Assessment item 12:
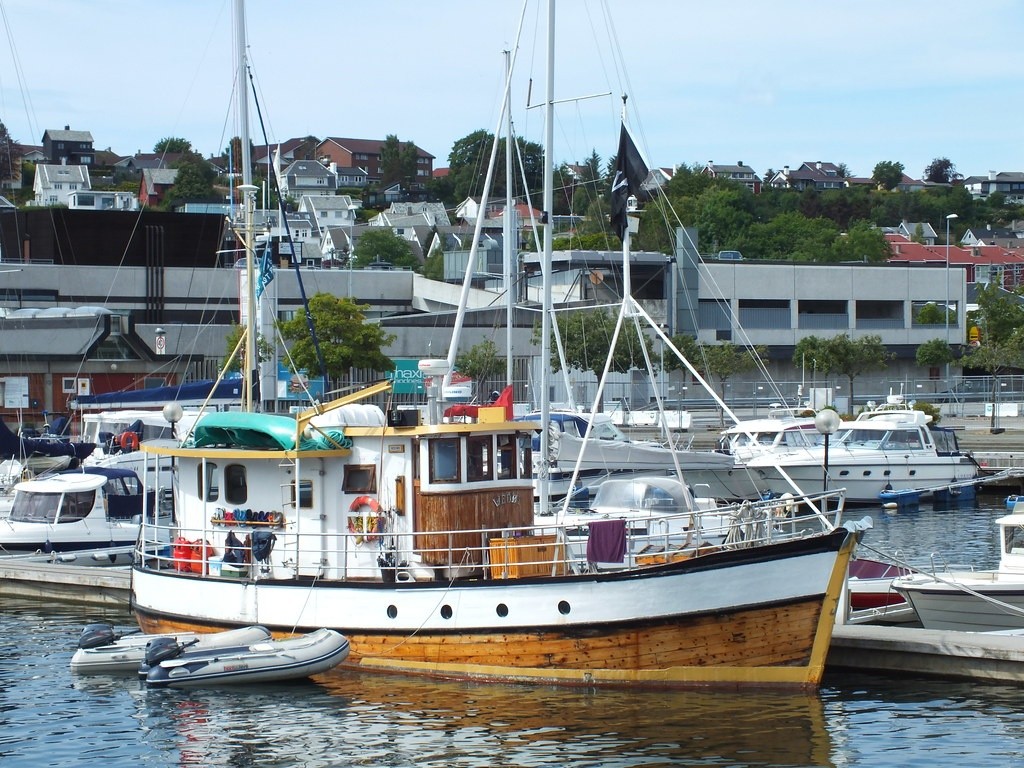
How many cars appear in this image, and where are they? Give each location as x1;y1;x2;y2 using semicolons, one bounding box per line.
715;250;747;261
934;379;995;403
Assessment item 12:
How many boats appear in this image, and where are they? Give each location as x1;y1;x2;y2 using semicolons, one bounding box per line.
0;0;1024;697
69;618;273;677
136;625;351;690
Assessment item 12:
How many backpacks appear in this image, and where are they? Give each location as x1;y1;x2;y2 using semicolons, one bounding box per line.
224;531;245;568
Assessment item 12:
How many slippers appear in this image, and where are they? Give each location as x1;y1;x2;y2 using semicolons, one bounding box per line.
214;507;283;530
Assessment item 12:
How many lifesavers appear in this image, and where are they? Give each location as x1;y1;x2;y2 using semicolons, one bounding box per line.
348;496;383;542
120;432;138;448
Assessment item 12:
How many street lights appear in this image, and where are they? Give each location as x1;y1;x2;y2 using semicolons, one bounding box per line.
946;213;958;418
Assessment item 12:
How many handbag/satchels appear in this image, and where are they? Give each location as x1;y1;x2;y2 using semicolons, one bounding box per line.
252;531;277;561
